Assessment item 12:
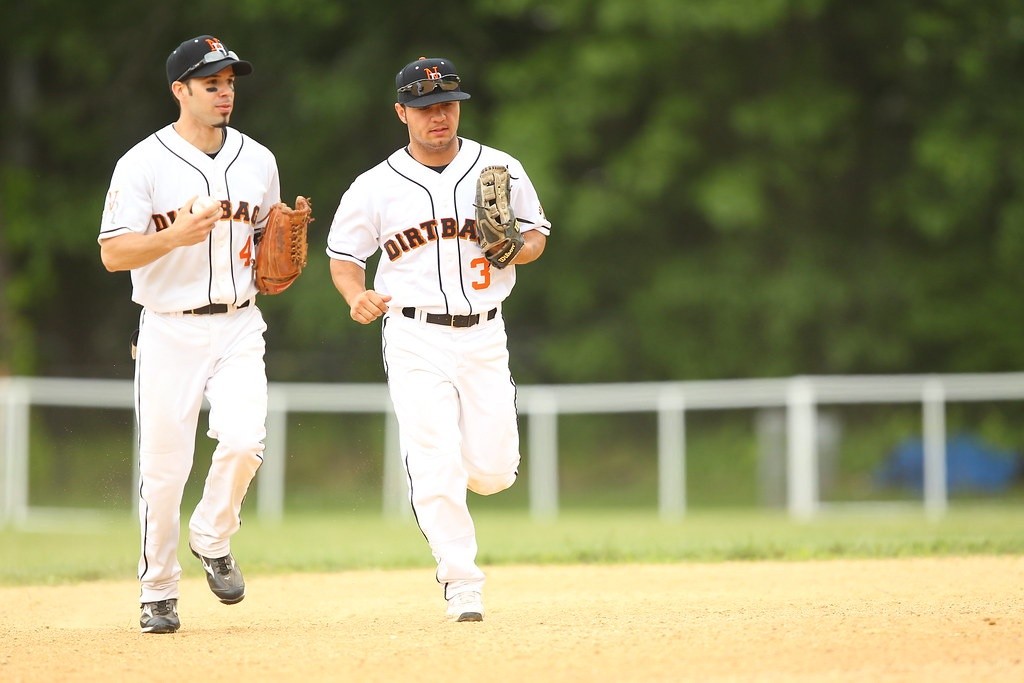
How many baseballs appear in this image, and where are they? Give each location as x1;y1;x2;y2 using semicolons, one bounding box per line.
191;195;221;220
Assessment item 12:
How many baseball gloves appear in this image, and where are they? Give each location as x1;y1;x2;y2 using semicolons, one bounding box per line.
473;164;525;271
255;195;314;296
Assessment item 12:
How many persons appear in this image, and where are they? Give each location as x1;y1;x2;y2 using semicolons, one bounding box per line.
325;56;552;631
95;36;315;635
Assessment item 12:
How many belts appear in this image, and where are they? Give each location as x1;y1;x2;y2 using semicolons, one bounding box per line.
401;306;498;328
182;298;251;315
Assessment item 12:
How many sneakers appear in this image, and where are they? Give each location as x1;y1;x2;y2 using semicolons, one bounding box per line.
138;591;181;635
189;538;248;606
443;578;489;624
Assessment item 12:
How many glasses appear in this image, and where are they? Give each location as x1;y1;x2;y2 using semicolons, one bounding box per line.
397;74;461;96
176;50;241;82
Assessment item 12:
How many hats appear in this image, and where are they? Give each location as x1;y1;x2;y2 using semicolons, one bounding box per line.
164;34;253;84
396;57;472;106
